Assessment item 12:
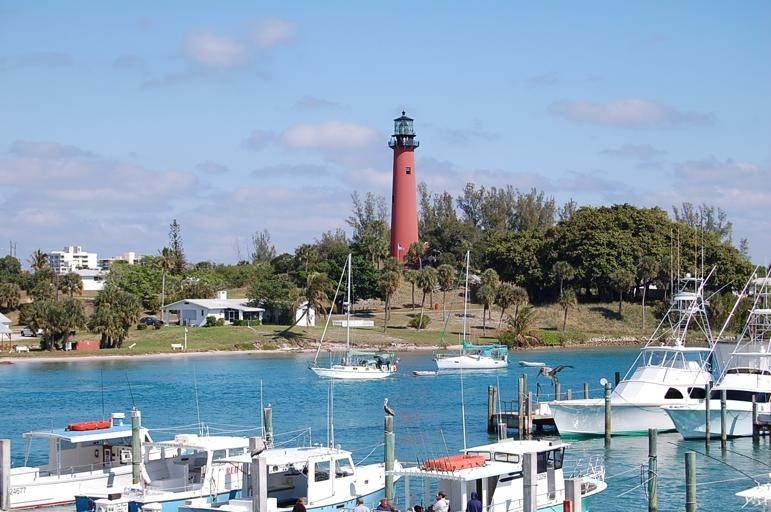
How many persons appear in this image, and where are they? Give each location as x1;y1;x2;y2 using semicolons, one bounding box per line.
466;491;482;511
292;498;306;512
376;358;383;369
413;491;450;511
354;497;371;512
377;498;393;512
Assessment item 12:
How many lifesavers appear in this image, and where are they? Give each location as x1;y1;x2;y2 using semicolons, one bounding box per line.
67;421;109;431
426;455;485;471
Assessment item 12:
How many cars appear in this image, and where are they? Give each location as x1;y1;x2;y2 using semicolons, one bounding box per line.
21;325;45;337
140;317;164;326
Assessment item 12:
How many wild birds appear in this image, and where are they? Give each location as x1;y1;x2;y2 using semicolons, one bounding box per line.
535;362;575;388
382;396;394;417
